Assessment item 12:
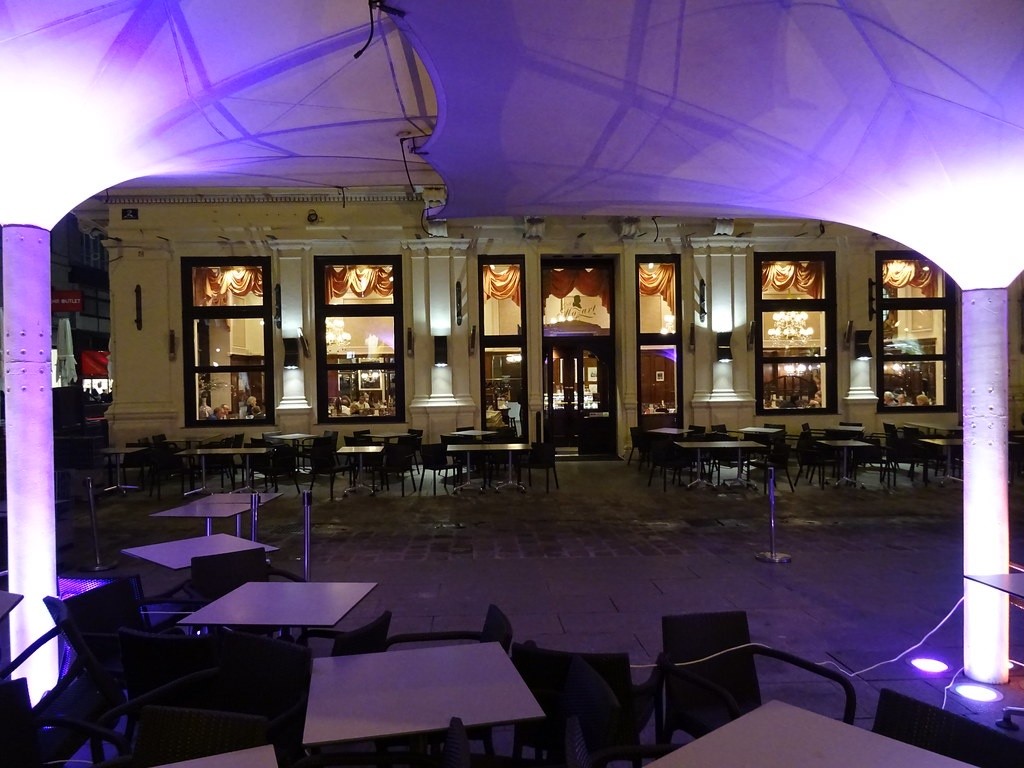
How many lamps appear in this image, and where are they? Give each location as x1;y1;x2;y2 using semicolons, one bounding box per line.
717;332;734;363
282;339;300;369
325;319;352;353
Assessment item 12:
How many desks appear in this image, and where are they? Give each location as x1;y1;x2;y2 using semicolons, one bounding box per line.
268;434;318;474
817;440;874;489
156;744;277;768
825;426;865;434
100;447;148;495
148;503;264;536
918;439;963;488
647;427;693;435
362;433;411;443
674;441;767;491
447;443;532;494
175;582;379;647
301;641;547;768
641;700;980;767
905;423;963;434
738;427;783;433
450;430;499;439
336;446;384;497
642;409;677;428
120;533;280;570
175;447;267;496
189;493;284;537
163;433;223;479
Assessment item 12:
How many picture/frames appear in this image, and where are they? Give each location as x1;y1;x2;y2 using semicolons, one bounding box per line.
358;358;383;391
587;367;597;382
337;359;357;392
559;359;577;384
656;371;664;382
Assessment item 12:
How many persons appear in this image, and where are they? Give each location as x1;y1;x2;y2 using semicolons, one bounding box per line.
206;396;266;419
83;388;113;403
327;395;370;417
763;390;822;408
884;391;929;406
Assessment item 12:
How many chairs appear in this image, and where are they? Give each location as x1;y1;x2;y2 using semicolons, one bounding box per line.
627;422;963;493
105;426;560;502
0;548;1024;767
